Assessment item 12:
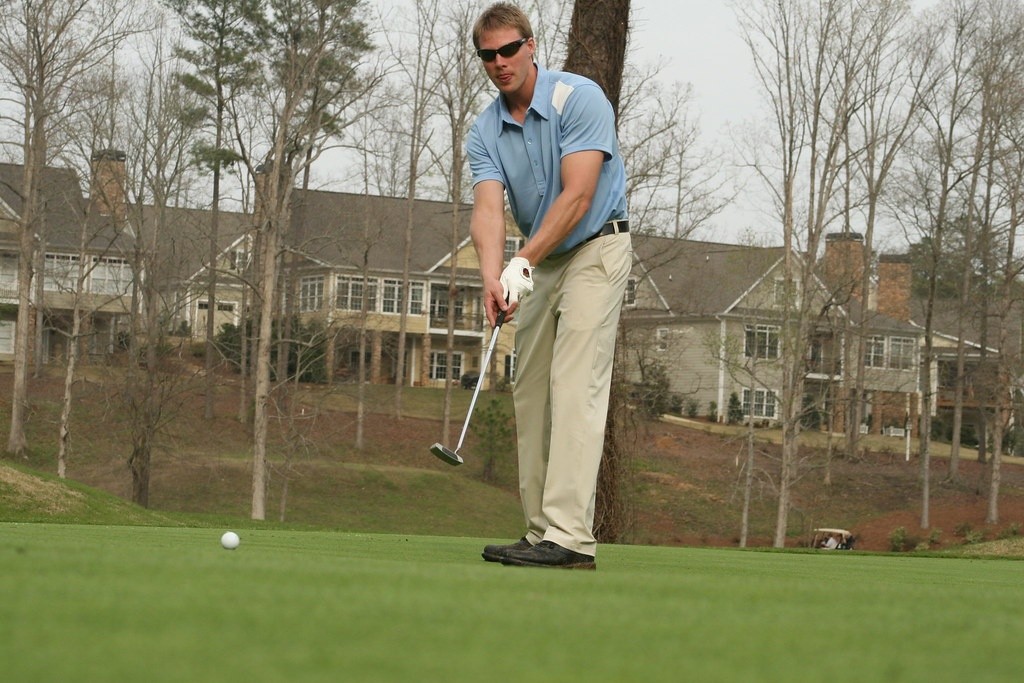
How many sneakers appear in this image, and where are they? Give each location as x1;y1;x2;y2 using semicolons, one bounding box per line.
481;536;533;562
499;540;596;570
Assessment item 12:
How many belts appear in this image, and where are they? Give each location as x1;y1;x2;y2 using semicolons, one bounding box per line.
586;220;629;242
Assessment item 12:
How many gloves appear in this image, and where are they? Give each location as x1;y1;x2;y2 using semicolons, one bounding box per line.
499;257;535;307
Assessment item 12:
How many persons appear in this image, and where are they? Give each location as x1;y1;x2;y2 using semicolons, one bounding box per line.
821;533;837;550
465;1;634;570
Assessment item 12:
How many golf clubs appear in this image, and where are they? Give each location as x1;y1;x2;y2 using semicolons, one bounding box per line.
430;293;511;465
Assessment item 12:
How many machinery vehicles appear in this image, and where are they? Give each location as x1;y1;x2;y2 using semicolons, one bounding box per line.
812;528;853;550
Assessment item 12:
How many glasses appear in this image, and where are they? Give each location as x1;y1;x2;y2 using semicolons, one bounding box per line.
477;37;527;62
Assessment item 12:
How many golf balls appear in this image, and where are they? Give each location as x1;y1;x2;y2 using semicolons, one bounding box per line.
221;531;240;550
